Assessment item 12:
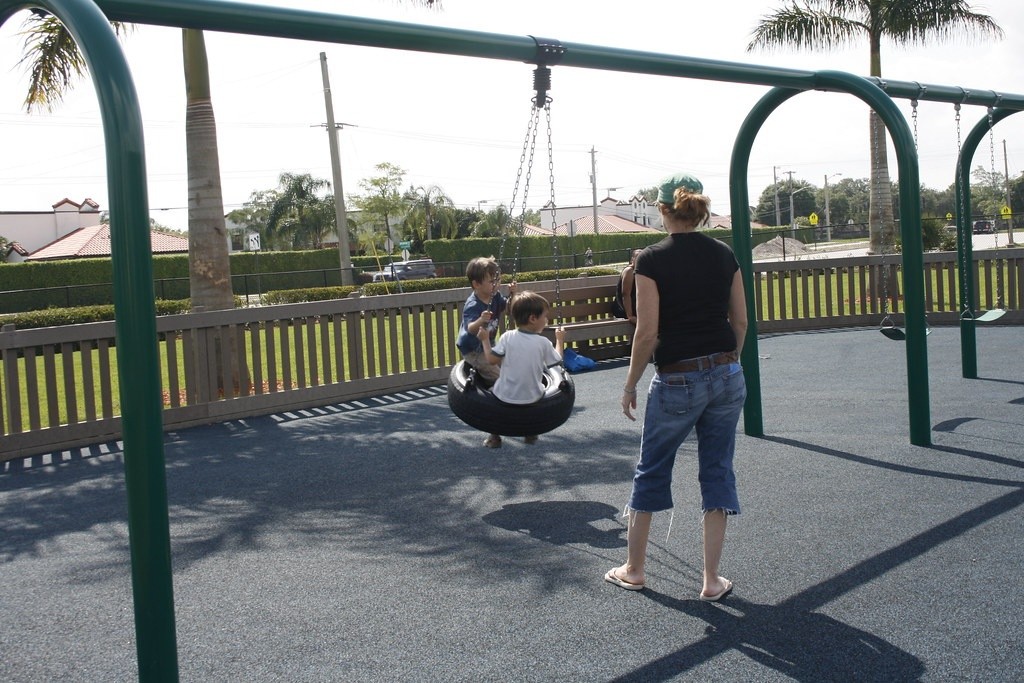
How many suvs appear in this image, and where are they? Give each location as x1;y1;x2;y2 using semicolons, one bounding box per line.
972;221;994;235
373;259;437;282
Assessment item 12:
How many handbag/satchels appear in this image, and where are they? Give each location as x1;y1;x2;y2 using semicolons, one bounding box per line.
562;348;596;372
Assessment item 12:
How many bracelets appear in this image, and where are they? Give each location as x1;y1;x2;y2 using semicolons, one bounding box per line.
621;382;638;393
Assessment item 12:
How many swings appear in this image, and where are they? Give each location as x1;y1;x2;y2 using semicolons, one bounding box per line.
446;63;576;438
868;97;933;342
954;102;1009;323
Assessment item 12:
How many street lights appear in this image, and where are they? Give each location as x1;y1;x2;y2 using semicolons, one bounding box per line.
823;172;842;242
478;200;487;212
788;185;809;239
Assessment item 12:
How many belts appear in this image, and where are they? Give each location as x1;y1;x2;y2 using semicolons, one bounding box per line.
659;350;738;372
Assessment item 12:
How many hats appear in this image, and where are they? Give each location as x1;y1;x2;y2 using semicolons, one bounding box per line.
657;172;703;204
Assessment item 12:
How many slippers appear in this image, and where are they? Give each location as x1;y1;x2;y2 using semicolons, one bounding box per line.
700;577;732;601
605;568;643;589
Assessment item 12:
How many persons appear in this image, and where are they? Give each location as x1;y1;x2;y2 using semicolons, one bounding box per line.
604;171;747;600
609;248;642;326
454;254;518;383
584;246;593;265
474;289;566;448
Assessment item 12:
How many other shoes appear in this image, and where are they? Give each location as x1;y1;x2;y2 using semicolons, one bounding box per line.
523;435;538;445
483;435;502;448
